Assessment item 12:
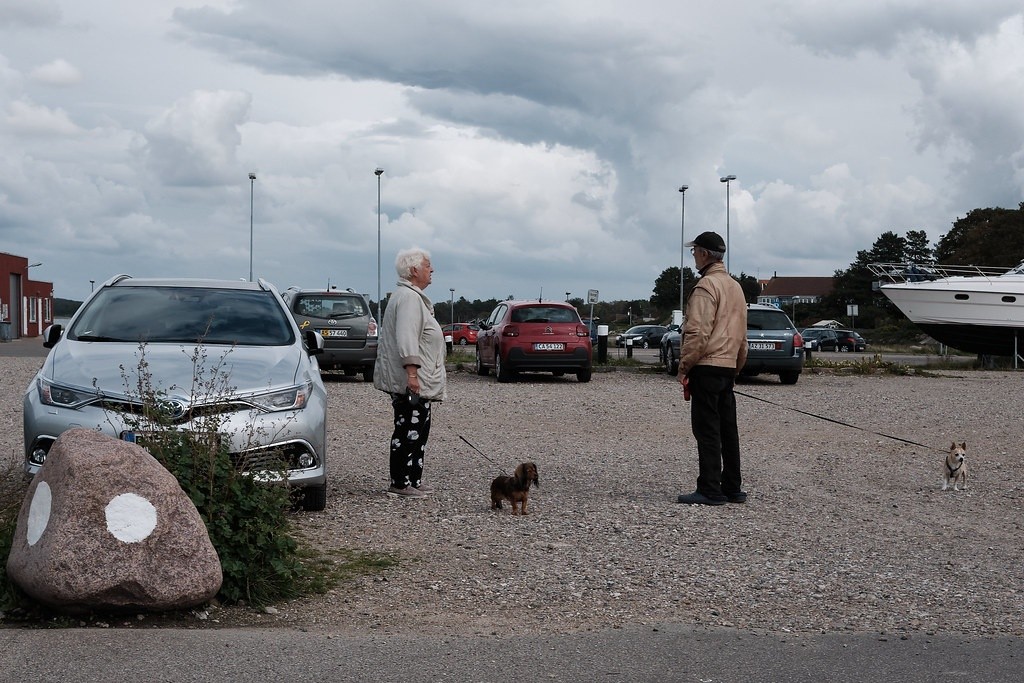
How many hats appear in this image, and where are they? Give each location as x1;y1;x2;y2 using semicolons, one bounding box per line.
684;232;726;253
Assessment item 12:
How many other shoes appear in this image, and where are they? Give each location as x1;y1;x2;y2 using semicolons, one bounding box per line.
417;484;434;491
724;494;746;502
678;492;725;505
388;484;424;496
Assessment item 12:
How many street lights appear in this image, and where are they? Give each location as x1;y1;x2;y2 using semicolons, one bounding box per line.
88;280;95;295
375;166;386;336
720;175;737;276
248;172;256;282
678;184;688;310
20;262;42;337
449;287;456;354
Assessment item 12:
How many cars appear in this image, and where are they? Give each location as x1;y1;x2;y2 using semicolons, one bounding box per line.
475;298;598;383
23;274;327;511
661;302;805;386
616;325;669;350
442;323;482;345
799;328;866;352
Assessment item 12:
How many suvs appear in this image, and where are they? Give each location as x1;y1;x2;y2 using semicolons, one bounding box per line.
277;286;378;382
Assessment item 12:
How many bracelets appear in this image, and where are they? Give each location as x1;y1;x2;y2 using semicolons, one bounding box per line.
409;375;417;378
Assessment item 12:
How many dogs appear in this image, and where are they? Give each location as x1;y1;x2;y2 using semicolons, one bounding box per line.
941;441;968;492
490;462;539;516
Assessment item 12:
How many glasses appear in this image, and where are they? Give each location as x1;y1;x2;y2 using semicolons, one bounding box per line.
690;248;700;254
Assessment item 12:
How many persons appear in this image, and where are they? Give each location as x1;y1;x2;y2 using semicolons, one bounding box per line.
373;247;446;497
675;232;748;503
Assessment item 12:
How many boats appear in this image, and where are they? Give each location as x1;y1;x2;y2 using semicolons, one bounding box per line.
867;257;1024;356
